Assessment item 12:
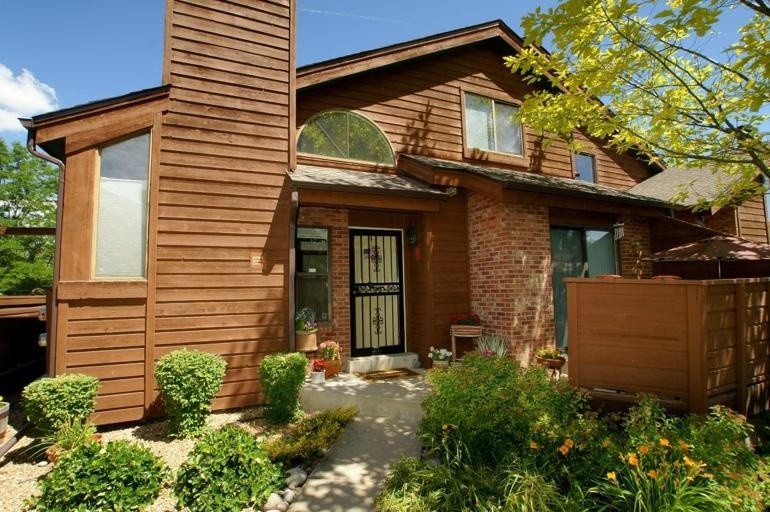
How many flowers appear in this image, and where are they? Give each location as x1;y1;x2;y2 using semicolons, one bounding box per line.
313;360;326;372
452;314;479;325
296;308;319;332
428;346;452;361
318;340;341;360
537;347;561;357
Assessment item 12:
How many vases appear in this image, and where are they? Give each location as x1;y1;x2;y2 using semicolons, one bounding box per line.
432;360;449;369
295;329;317;353
311;372;326;384
536;358;565;370
323;360;341;377
